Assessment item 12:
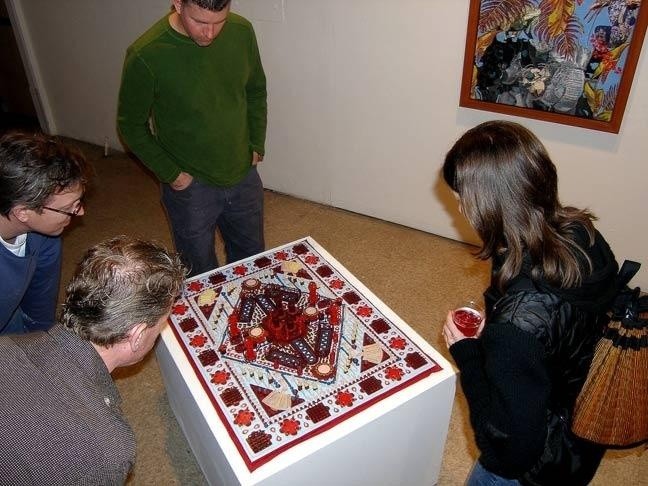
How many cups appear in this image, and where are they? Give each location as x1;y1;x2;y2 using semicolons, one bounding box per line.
452;302;484;337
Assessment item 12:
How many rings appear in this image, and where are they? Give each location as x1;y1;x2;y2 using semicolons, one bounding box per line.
440;330;446;336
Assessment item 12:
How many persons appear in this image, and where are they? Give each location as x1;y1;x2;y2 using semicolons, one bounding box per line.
0;131;95;337
1;235;191;486
440;117;647;486
113;0;270;277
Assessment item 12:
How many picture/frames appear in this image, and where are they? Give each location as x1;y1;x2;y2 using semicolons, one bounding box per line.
458;0;648;135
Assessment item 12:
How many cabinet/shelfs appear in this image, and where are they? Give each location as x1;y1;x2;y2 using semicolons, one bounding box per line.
154;236;459;486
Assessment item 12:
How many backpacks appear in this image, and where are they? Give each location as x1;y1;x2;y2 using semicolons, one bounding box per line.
531;227;648;451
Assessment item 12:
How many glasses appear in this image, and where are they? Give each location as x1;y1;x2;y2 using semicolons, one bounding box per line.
40;201;83;219
